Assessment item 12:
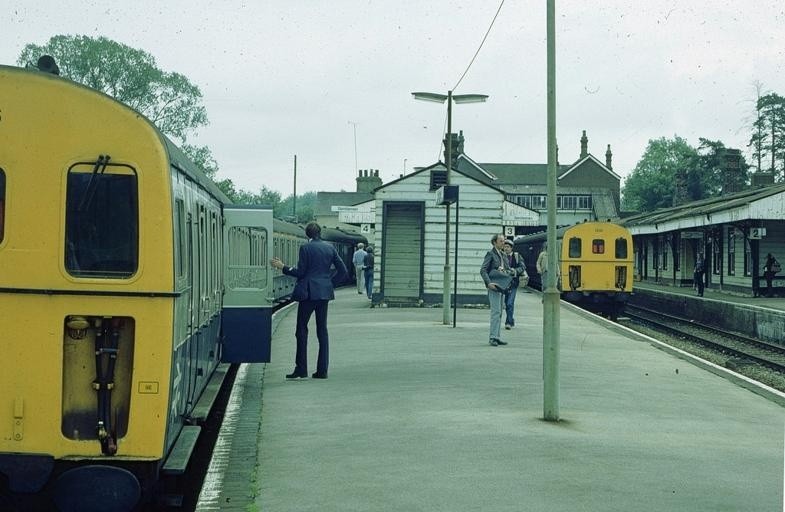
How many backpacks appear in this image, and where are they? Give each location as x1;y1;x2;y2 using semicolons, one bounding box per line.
514;252;530;289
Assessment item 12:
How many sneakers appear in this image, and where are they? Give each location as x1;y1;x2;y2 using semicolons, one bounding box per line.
505;322;514;331
489;339;508;346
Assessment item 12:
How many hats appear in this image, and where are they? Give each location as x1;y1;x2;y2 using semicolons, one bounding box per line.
505;240;514;246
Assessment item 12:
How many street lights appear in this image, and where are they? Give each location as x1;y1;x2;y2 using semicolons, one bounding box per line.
407;85;494;324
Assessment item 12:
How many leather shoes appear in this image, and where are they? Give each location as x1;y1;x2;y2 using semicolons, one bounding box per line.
285;369;308;379
312;371;330;379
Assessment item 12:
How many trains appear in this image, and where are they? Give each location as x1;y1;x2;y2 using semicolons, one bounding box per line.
1;48;379;512
512;217;637;321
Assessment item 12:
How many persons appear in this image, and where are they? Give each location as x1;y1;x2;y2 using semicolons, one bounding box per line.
266;222;347;379
535;241;559;303
351;241;368;294
762;252;780;295
501;238;528;329
479;233;508;347
694;252;708;298
364;246;375;300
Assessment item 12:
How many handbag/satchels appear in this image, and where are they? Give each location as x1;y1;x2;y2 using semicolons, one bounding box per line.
486;269;514;294
